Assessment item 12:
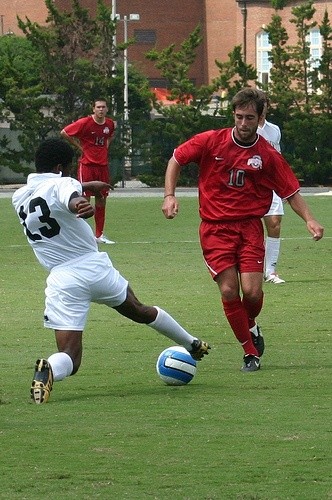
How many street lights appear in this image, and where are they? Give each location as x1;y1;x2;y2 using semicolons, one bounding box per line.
111;13;141;172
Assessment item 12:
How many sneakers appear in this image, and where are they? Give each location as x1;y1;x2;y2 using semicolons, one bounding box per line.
243;354;260;372
248;321;264;357
187;338;211;361
95;234;115;245
30;359;53;405
265;273;285;284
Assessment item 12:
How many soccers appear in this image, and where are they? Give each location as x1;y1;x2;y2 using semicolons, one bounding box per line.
156;346;196;385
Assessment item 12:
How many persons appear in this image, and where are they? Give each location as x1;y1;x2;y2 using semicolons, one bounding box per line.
60;98;116;245
12;140;211;405
162;88;325;371
256;96;285;284
